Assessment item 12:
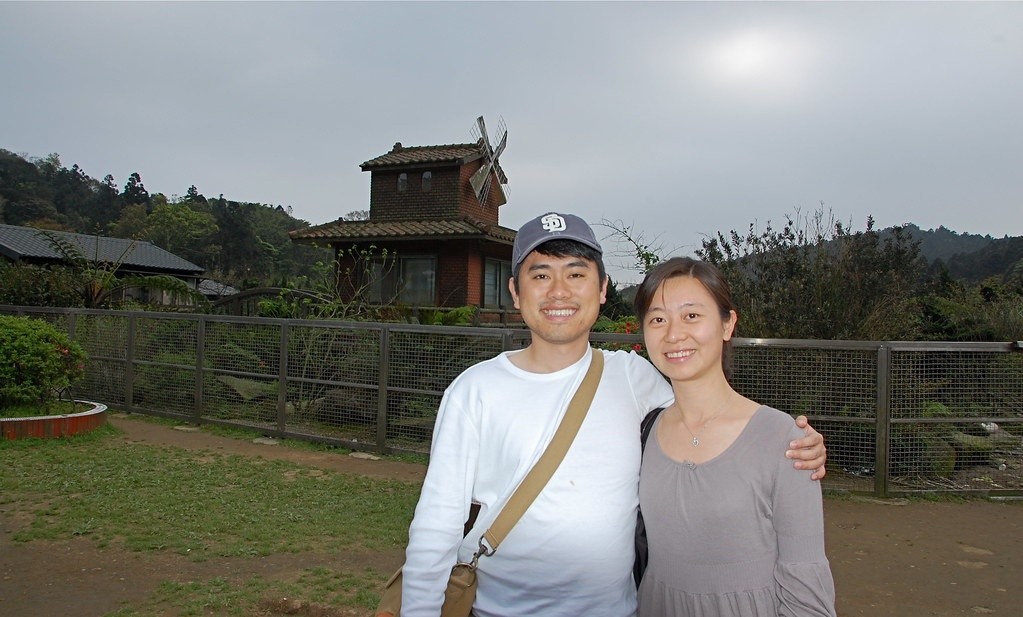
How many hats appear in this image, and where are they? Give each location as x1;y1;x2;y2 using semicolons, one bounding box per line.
512;212;604;275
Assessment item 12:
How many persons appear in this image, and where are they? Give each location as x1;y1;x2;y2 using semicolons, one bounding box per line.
397;212;825;617
637;257;837;617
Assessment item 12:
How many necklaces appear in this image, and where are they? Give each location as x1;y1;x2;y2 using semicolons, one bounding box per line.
673;405;723;447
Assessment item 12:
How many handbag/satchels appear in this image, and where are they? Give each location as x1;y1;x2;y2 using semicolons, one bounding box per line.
633;407;665;592
375;548;478;617
